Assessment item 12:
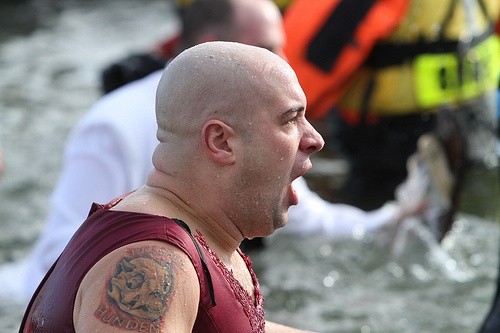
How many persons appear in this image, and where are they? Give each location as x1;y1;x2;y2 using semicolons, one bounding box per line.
149;1;500;243
30;0;289;296
19;40;325;333
